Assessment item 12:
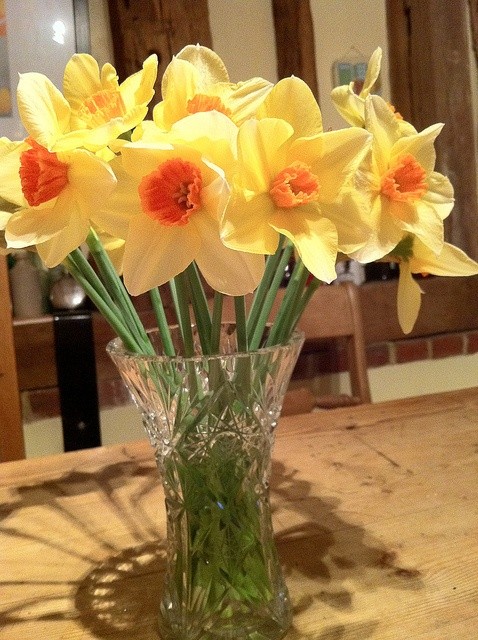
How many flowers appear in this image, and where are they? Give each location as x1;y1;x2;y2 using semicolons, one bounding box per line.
0;43;478;627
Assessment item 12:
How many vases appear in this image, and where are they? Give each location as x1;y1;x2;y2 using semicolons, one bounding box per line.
107;325;303;639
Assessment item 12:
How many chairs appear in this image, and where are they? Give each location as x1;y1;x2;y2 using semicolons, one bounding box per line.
186;278;372;418
9;310;102;452
0;249;26;463
